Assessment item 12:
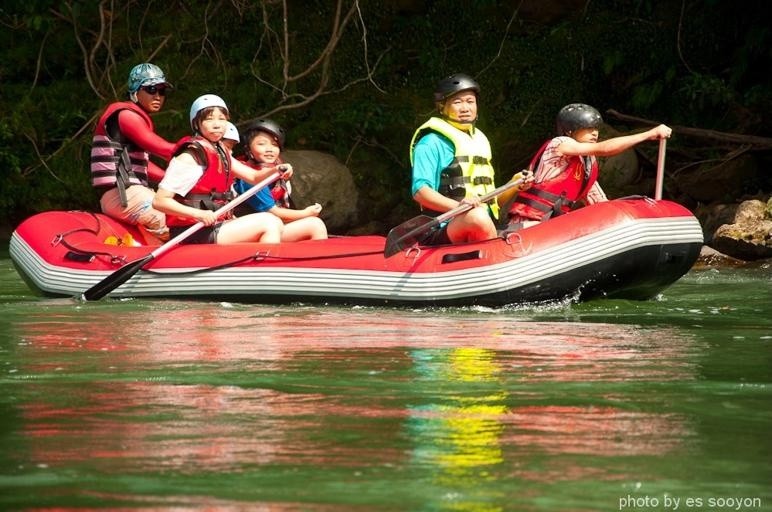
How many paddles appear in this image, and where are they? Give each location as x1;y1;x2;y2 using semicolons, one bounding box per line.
383;169;529;258
82;165;289;303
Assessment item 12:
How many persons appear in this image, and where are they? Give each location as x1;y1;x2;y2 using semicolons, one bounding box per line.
232;117;323;224
90;63;177;242
409;71;534;247
507;102;673;229
151;93;329;244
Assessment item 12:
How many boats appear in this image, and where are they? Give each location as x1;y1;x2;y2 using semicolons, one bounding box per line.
8;193;705;309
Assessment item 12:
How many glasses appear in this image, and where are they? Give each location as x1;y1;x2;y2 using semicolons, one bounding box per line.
139;86;166;96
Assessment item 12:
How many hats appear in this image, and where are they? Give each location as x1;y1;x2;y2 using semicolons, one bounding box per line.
222;120;241;143
435;74;478;99
560;104;603;132
248;118;286;147
192;95;227;129
127;63;173;92
